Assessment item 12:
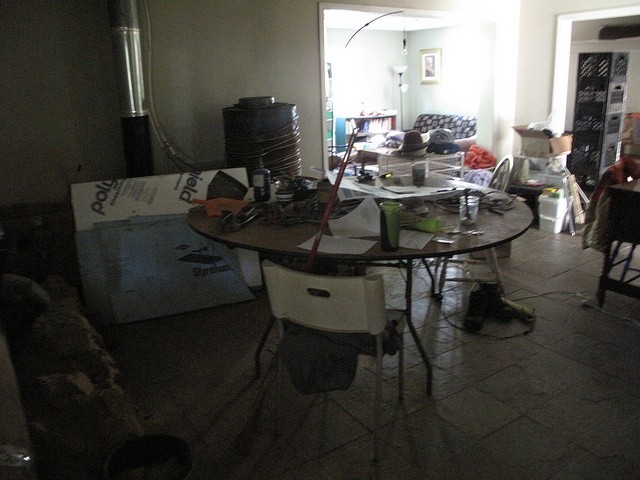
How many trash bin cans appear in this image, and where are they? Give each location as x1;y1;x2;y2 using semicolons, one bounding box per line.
102;433;192;480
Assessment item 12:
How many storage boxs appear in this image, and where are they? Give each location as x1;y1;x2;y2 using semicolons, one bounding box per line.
529;155;567;173
538;196;571;234
512;124;573;157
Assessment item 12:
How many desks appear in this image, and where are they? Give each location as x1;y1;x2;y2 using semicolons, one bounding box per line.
596;176;640;310
353;142;465;178
186;171;534;376
343;108;397;131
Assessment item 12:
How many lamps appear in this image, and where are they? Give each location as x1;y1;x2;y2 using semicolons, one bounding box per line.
393;65;409;132
401;27;407;56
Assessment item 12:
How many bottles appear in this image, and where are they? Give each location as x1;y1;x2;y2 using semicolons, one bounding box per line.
253;163;271;200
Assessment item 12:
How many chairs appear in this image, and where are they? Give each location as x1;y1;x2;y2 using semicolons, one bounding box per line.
261;260;406;463
430;155;517;296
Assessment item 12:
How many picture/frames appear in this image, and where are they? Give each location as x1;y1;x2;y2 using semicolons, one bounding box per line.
418;49;442;83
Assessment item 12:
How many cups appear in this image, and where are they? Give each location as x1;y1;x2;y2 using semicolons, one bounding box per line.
379;201;403;251
412;158;428;187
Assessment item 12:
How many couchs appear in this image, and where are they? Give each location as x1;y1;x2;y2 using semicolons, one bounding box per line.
403;114;477;153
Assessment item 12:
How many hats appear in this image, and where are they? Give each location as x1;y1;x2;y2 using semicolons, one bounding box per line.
392;132;429;153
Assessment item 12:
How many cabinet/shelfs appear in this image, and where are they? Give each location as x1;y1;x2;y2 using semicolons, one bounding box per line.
325;62;336;157
570;51;628;190
514;185;552;229
